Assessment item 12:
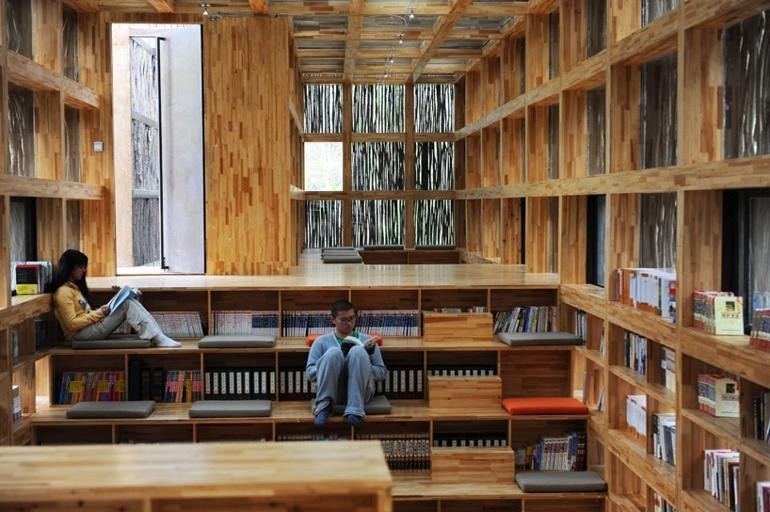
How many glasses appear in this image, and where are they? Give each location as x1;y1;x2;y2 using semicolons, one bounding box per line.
334;316;358;324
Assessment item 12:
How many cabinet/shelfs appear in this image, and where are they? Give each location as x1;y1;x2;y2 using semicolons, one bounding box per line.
128;34;170;272
453;0;770;512
0;0;116;294
303;77;456;251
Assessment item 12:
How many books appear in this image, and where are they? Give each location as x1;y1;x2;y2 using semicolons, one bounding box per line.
352;306;556;401
12;330;23;422
16;261;53;295
595;265;770;511
274;431;585;474
59;310;334;404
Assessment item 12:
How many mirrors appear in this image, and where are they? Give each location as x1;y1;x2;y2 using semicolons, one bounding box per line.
111;22;206;277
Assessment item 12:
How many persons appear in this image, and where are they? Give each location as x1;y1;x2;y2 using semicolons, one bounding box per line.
45;248;182;348
